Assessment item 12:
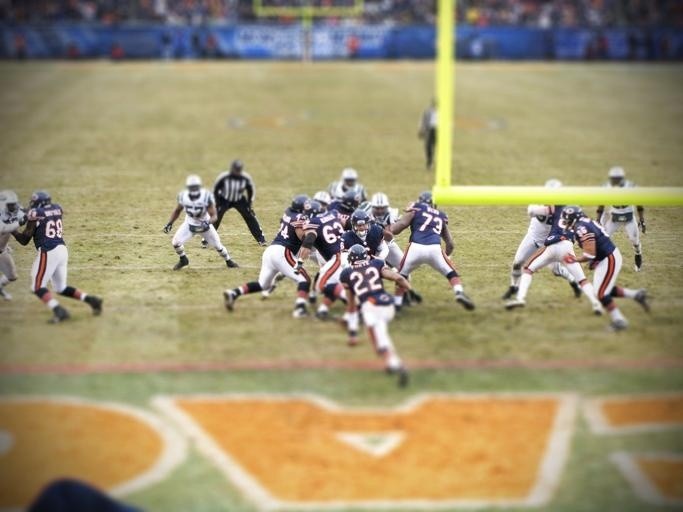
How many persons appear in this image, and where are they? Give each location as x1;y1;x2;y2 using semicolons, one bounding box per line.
0;190;43;302
501;166;657;332
10;190;104;325
415;97;439;169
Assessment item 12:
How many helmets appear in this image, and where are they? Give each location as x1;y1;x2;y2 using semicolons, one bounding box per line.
340;167;357;181
369;191;379;207
346;242;367;268
415;191;437;208
342;190;360;207
29;190;52;210
184;174;202;198
349;209;371;237
559;205;584;229
288;190;333;218
0;188;20;217
607;165;625;179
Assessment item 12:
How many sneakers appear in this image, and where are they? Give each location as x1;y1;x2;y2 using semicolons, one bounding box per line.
200;237;209;249
45;305;72;325
171;255;189;273
83;295;103;318
632;287;653;316
568;279;581;299
454;290;475;312
592;301;627;330
0;287;13;301
257;239;267;247
500;284;526;311
224;259;239;269
291;304;328;321
223;288;237;313
632;252;643;272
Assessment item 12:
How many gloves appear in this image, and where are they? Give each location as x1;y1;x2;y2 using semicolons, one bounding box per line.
162;222;173;234
247;205;256;217
199;220;210;230
563;253;575;266
637;220;648;234
294;257;304;275
407;288;421;305
